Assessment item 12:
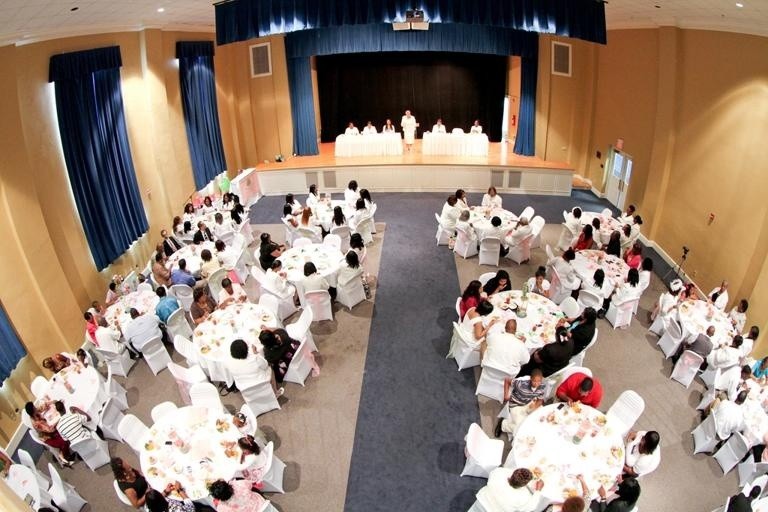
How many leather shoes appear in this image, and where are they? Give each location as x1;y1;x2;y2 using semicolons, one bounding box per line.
58;455;76;468
276;386;285;400
494;418;504;437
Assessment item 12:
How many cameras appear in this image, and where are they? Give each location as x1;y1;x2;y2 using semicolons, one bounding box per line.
683;246;689;254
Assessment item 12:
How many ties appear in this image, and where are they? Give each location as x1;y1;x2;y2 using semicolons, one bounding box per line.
169;240;175;251
203;233;208;241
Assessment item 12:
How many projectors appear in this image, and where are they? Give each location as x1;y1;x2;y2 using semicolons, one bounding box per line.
407;10;423;22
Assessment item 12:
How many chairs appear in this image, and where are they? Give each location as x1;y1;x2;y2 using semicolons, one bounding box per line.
451;127;464;134
431;180;765;512
1;168;379;512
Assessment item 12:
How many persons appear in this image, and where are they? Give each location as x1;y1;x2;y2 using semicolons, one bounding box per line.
343;110;483;153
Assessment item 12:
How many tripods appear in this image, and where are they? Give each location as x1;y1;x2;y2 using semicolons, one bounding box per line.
661;254;686;281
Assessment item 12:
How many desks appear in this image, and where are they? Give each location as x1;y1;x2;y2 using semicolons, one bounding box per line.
421;128;488;157
332;131;404;159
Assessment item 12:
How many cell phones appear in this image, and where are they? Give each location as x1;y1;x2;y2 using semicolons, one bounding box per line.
558;403;564;410
165;441;172;445
